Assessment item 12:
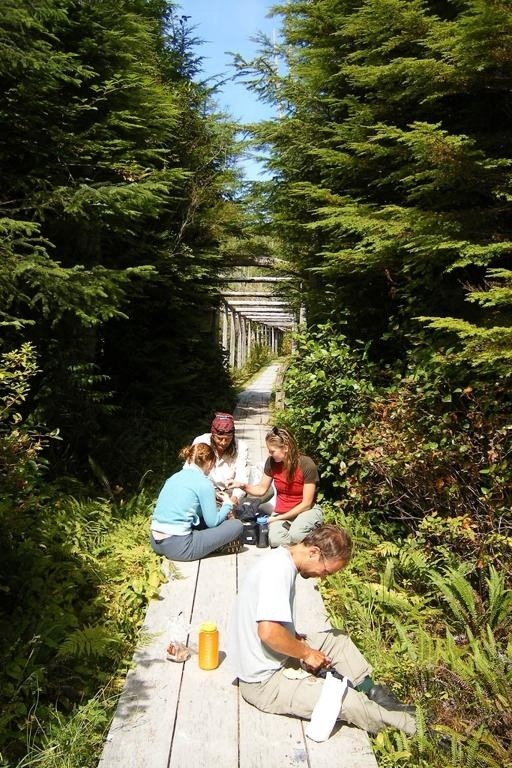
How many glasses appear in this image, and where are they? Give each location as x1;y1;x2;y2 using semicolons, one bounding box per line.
273;427;283;441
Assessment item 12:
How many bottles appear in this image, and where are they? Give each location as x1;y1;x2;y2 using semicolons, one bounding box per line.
256;515;270;548
198;621;220;670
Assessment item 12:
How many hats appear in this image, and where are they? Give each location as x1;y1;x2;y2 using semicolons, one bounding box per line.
212;413;234;435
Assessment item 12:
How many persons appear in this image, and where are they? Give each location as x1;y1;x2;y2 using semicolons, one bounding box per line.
230;524;419;739
225;427;324;547
149;443;245;562
192;413;276;515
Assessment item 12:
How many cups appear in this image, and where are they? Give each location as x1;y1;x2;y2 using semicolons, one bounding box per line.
243;521;258;544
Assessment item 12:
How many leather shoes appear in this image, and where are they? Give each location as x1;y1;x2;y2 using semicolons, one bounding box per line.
369;684;416;711
